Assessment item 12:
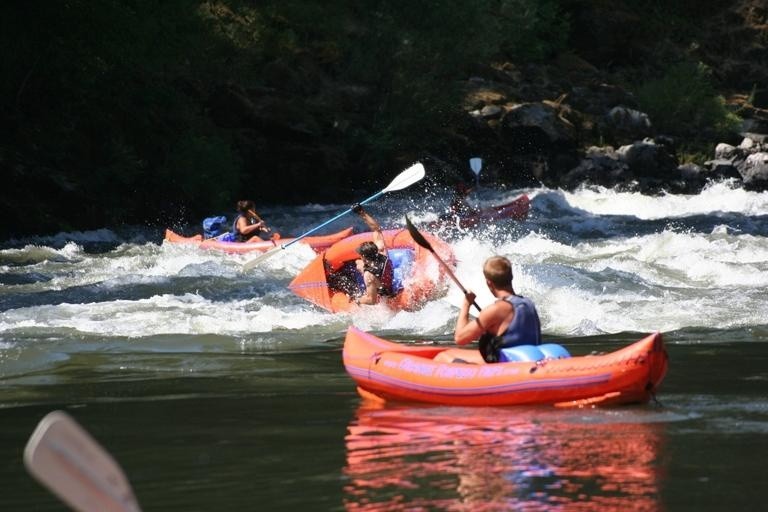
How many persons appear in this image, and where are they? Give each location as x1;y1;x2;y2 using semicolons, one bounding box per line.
434;254;542;366
233;198;272;242
450;177;483;221
330;203;396;305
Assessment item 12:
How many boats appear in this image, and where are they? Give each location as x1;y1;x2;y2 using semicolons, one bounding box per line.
162;220;358;252
423;197;540;229
288;229;460;312
342;323;668;405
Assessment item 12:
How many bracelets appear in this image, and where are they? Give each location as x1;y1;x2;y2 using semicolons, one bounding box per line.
257;221;262;228
355;297;360;305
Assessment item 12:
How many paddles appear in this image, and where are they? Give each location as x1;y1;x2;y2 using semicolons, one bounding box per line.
241;162;426;270
469;159;482;201
23;409;138;512
403;210;483;312
